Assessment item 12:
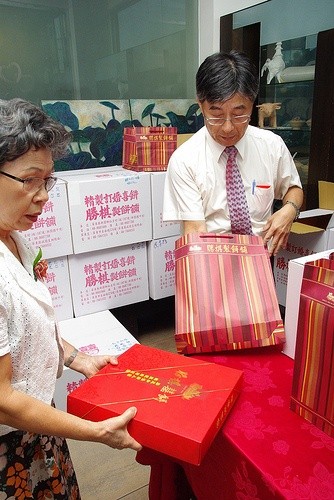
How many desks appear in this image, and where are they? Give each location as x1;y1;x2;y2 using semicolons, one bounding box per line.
135;344;334;500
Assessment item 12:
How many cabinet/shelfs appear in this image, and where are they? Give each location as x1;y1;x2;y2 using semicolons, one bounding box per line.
256;33;317;188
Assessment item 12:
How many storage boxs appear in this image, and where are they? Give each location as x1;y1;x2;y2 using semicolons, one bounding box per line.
17;163;181;414
67;344;246;466
273;181;334;359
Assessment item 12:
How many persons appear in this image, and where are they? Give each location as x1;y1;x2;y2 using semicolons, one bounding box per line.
0;98;143;500
160;51;304;290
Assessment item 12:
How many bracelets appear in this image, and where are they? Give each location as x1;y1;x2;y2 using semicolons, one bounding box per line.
282;201;300;223
64;347;78;367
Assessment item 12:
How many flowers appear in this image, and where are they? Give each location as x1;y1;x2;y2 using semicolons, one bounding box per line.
32;247;48;283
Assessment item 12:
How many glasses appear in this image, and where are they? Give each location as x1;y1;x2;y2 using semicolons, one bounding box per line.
201;102;253;126
0;170;57;194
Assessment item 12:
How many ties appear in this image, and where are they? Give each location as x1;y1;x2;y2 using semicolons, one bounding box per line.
224;146;253;235
